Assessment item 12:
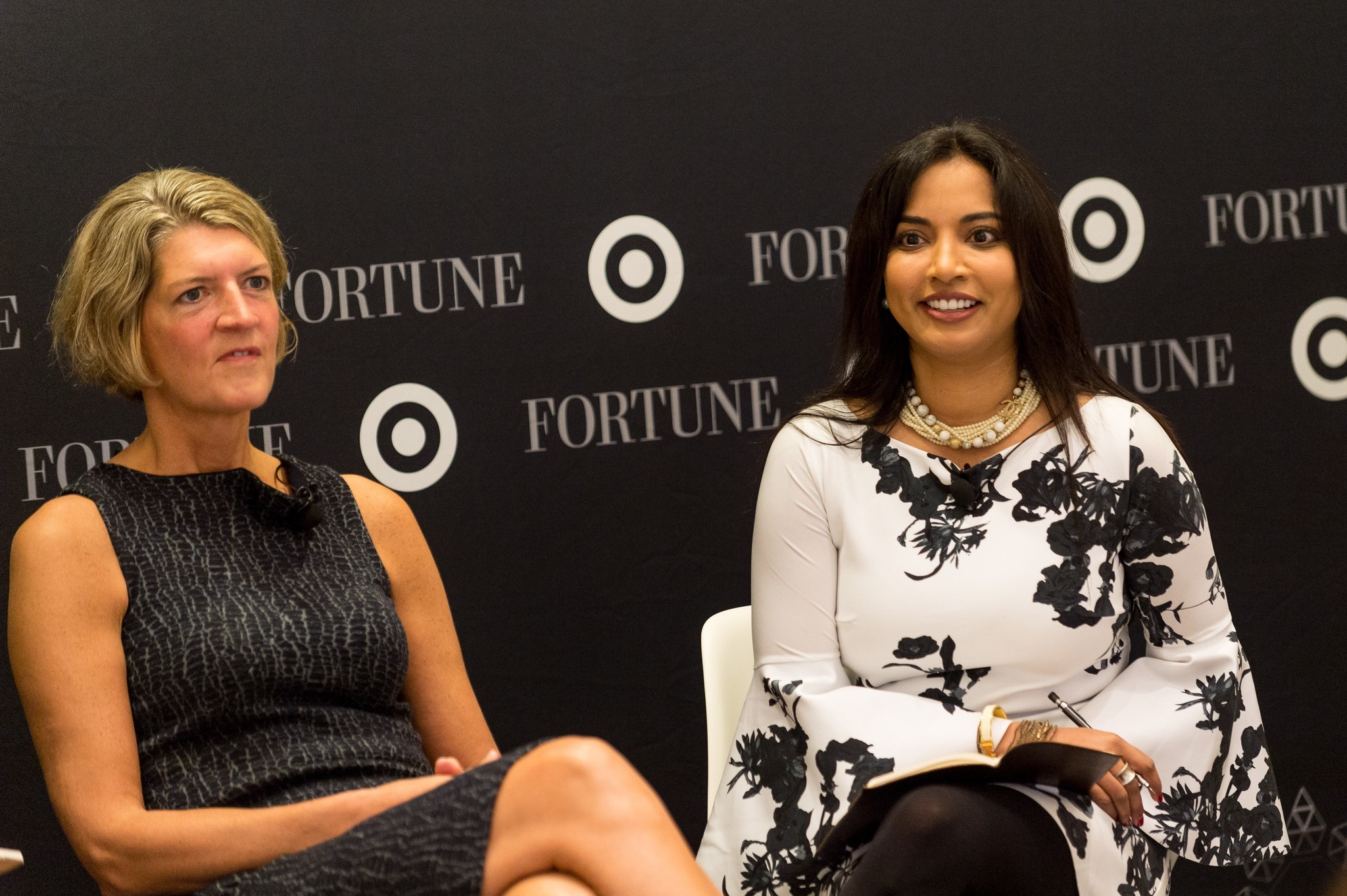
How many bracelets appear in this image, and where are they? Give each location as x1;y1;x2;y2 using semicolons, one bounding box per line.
979;703;1007;755
1007;720;1057;751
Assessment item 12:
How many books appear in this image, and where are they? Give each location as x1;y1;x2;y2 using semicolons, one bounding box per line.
812;741;1124;863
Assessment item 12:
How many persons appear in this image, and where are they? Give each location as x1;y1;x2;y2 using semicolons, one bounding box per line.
695;120;1290;896
0;168;721;896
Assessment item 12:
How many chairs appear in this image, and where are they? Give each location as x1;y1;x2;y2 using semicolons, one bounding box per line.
701;604;755;821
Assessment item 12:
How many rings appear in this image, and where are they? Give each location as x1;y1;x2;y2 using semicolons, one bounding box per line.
1115;763;1137;786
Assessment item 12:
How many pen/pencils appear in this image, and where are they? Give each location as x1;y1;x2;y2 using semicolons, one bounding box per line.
1048;691;1156;795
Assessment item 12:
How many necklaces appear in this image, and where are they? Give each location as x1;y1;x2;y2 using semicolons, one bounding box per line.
898;371;1041;451
